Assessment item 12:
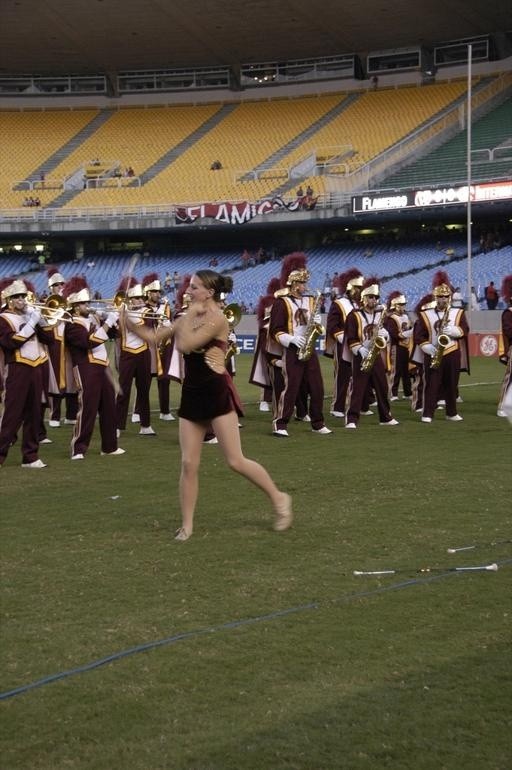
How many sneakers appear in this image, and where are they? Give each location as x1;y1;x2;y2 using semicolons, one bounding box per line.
21;410;176;469
259;394;464;436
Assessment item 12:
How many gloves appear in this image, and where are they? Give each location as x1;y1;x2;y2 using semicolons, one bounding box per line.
378;328;389;338
105;312;120;328
443;326;461;337
26;307;42;328
290;336;306;349
359;347;369;359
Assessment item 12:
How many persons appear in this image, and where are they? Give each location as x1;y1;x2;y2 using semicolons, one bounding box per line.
1;226;512;285
121;270;293;542
2;266;244;466
307;186;313;195
249;252;469;437
497;276;512;426
297;186;303;196
22;159;134;207
210;160;221;170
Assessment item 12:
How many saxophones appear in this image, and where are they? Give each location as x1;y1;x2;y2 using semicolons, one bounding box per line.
430;302;450;370
360;301;388;374
297;287;326;362
225;331;240;361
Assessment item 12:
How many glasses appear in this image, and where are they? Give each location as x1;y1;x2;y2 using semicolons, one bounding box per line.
11;294;27;299
439;295;449;298
368;295;380;299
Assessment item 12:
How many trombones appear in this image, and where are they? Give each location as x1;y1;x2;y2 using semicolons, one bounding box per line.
24;294;74;326
218;303;242;329
86;289;169;320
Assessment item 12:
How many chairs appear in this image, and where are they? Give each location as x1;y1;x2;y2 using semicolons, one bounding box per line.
0;73;511;214
0;235;512;309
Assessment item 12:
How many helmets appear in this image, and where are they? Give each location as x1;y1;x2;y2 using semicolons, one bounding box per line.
128;284;144;298
434;284;450;296
1;281;28;299
67;288;90;304
145;281;161;291
390;295;406;310
286;271;309;286
346;275;379;301
48;273;65;287
